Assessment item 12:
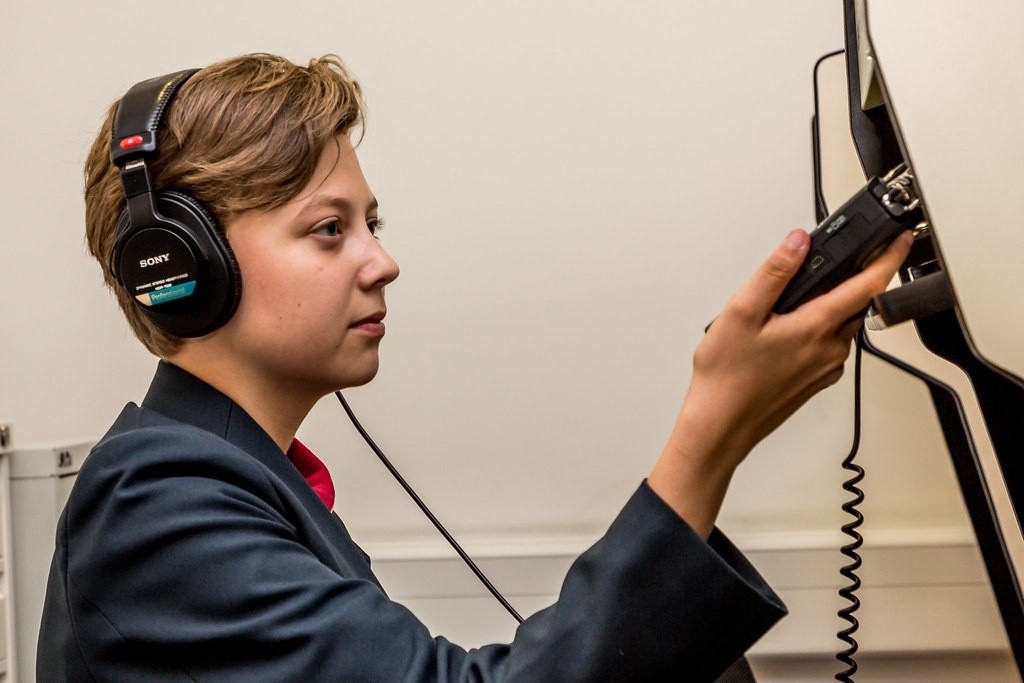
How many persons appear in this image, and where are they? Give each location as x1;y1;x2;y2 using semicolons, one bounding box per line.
36;53;913;682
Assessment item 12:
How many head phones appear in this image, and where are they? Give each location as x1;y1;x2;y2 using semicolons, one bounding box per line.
110;69;241;337
